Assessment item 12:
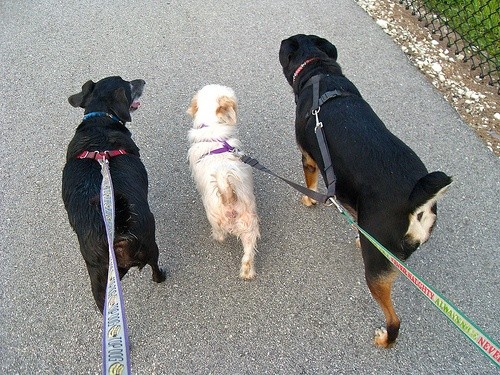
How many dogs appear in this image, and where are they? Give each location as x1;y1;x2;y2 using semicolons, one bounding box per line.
279;33;456;350
185;83;264;279
60;75;168;317
